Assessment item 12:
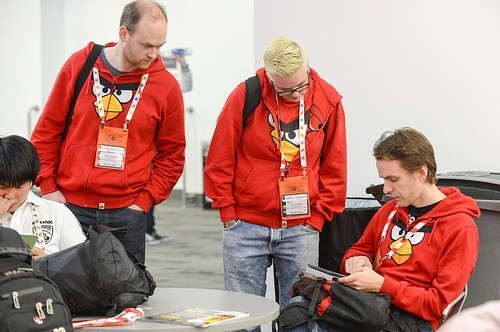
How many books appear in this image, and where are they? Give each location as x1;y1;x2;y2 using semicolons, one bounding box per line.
141;309;249;328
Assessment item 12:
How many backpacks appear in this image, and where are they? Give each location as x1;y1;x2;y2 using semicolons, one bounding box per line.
0;227;74;332
32;224;157;317
272;271;391;332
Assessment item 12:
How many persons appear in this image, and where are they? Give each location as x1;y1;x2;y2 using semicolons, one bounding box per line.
30;0;186;265
0;135;88;261
204;38;348;332
282;127;481;332
145;52;192;247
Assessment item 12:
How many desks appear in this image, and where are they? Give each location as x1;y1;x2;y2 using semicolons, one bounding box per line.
73;287;281;332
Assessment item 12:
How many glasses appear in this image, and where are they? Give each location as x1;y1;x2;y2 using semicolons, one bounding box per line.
271;71;310;97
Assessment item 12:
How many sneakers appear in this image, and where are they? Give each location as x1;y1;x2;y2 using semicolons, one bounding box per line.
145;231;170;246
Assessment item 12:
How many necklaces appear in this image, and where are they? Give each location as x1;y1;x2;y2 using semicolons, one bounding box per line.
0;202;47;253
